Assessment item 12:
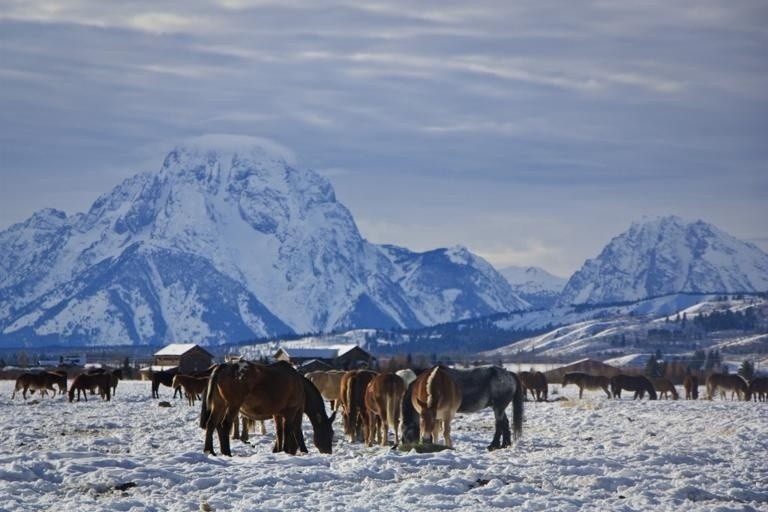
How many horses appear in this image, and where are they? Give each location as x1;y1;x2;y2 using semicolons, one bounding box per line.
683;375;700;400
152;371;182;399
173;373;208;405
202;359;336;455
519;371;548;401
304;360;524;454
29;370;68;395
67;366;123;403
11;372;67;400
562;371;611;399
611;375;657;400
648;377;679;400
706;373;768;402
